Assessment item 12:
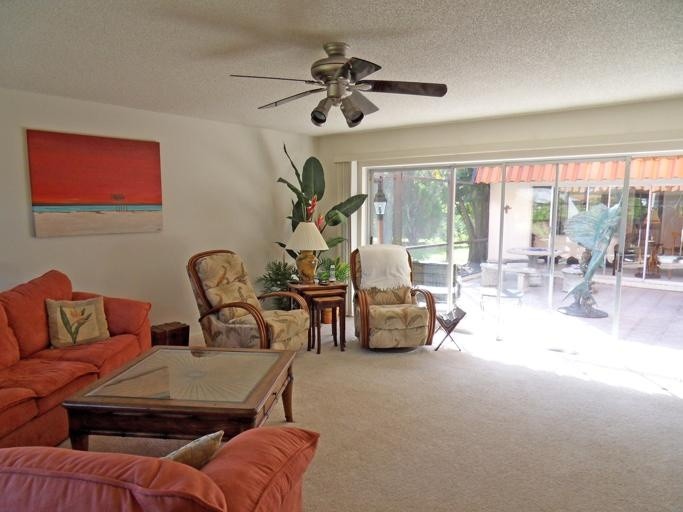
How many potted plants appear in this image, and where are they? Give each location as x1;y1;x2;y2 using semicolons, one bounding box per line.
315;258;348;324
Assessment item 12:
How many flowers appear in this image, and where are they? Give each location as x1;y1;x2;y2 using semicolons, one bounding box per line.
271;140;371;261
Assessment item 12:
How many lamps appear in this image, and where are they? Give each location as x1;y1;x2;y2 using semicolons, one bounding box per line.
283;221;328;282
641;205;662;241
304;84;365;129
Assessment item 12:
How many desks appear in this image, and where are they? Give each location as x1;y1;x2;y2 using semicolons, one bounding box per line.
286;281;350;355
508;246;561;286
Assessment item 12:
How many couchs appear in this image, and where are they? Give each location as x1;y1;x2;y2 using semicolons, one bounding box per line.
0;266;152;447
0;424;320;511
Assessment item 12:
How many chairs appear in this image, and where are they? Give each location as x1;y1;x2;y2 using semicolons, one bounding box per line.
346;243;434;352
185;247;313;356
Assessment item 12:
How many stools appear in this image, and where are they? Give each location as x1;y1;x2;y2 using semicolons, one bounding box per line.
433;304;468;351
479;258;589;292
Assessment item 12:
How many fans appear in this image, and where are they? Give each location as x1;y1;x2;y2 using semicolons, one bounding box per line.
226;41;449;112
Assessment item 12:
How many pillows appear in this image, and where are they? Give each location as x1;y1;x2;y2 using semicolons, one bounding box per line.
46;294;112;351
156;428;225;471
